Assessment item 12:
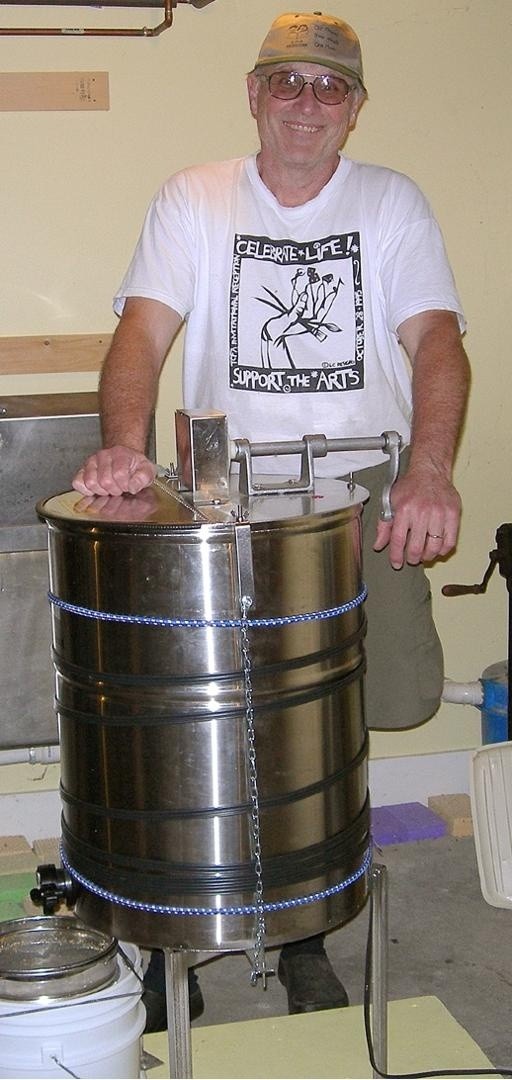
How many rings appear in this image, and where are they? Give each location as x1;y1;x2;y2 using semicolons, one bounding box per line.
428;534;444;539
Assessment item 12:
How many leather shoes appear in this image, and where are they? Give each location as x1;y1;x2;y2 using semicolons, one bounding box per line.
140;973;206;1037
276;950;350;1017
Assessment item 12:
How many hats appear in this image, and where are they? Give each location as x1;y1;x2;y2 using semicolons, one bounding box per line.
245;10;369;94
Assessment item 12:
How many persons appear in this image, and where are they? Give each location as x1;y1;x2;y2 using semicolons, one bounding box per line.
71;11;472;1032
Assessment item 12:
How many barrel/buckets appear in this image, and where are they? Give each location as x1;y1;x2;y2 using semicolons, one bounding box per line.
37;473;371;954
0;916;147;1079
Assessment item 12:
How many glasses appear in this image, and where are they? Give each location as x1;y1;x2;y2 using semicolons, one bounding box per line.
254;69;358;109
